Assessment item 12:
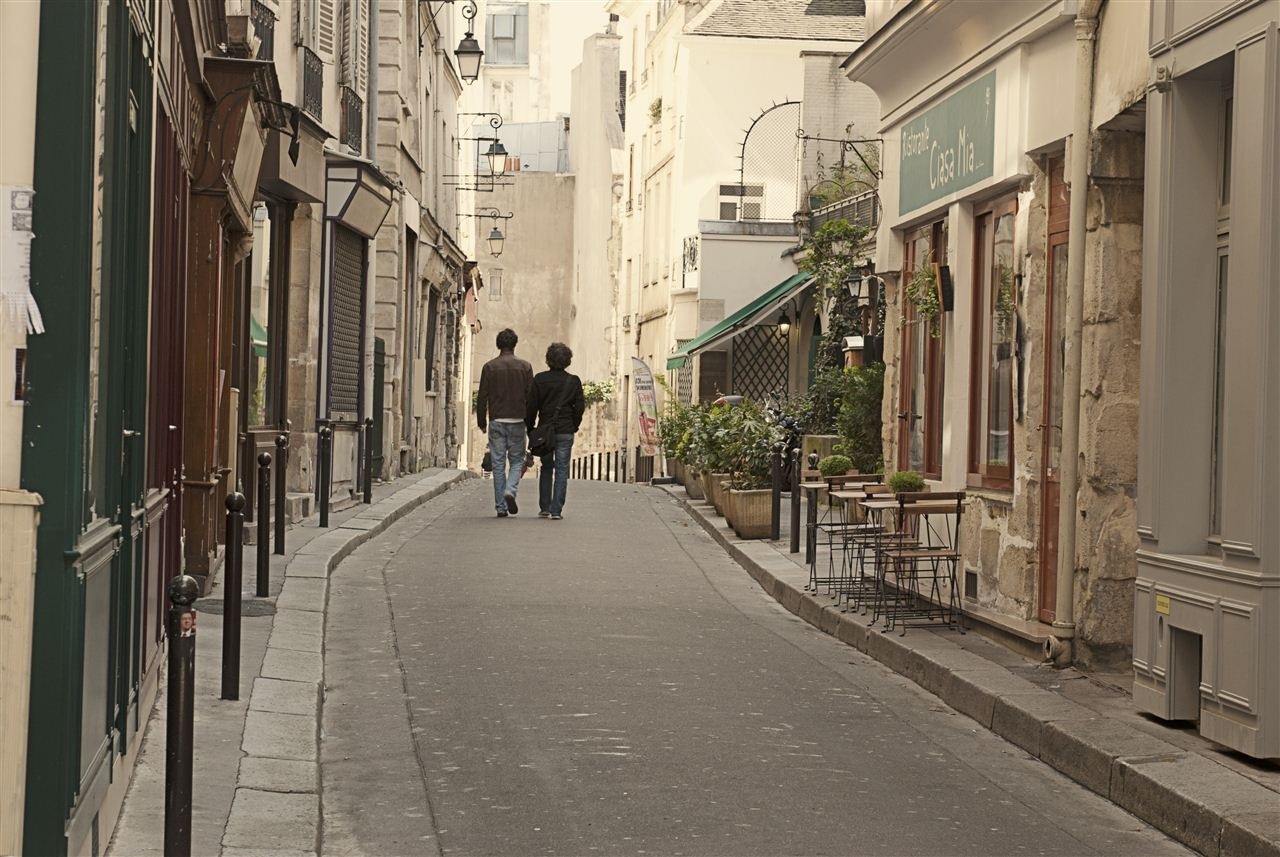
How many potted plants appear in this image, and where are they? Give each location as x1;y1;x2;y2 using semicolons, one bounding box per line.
650;372;789;539
817;454;854;485
886;472;925;504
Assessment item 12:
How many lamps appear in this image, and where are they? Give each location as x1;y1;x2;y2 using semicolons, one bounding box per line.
254;90;302;166
778;295;800;337
456;206;513;257
842;269;885;318
417;0;484;85
456;113;508;179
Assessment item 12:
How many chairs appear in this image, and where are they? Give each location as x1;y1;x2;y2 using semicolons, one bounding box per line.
816;477;967;637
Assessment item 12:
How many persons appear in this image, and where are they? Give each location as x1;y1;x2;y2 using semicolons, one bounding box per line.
476;328;534;517
524;342;584;520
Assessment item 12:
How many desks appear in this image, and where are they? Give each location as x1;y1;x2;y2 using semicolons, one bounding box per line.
797;482;970;634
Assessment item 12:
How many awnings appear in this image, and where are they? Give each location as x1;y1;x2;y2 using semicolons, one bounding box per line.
666;267;815;369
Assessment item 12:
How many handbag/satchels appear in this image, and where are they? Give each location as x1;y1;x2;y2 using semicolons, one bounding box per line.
530;420;573;457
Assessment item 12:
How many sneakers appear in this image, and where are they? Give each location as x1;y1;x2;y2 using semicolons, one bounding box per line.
536;510;551;517
553;513;564;520
497;511;508;517
505;492;518;515
